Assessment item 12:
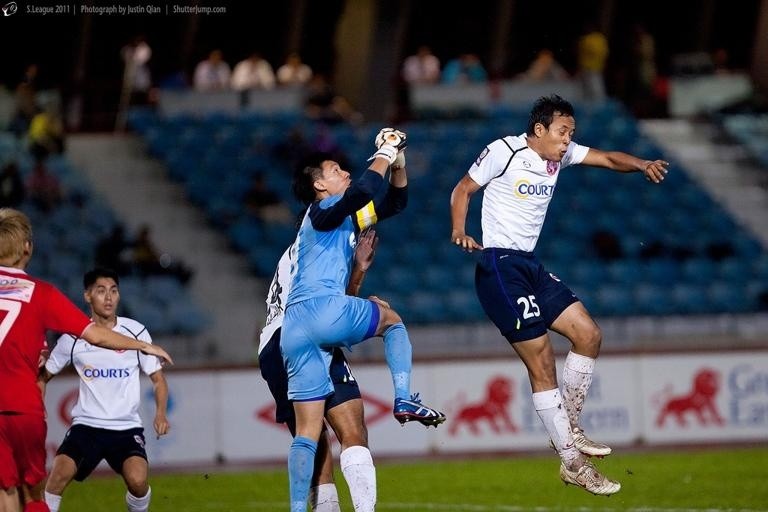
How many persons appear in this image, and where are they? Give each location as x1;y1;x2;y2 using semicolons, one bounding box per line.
276;127;447;512
258;206;380;512
2;207;176;511
44;265;172;511
451;92;670;491
1;20;668;287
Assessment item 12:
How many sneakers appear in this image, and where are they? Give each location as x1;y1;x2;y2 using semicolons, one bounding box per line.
393;393;447;429
549;427;613;459
559;461;622;497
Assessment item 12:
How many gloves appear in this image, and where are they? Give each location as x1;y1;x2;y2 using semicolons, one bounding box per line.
367;128;408;168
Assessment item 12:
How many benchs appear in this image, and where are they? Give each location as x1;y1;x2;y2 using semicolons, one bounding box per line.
6;95;764;346
